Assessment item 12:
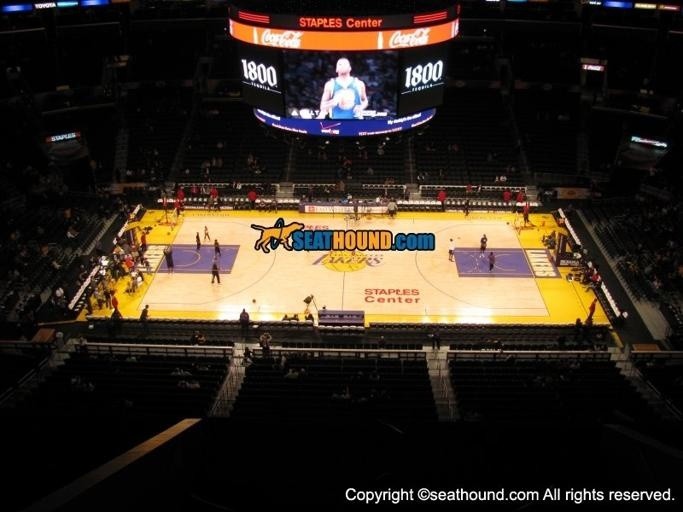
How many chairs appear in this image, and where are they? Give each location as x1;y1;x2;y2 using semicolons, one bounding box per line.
0;348;683;422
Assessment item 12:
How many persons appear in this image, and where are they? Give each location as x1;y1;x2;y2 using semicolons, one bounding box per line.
0;0;682;427
